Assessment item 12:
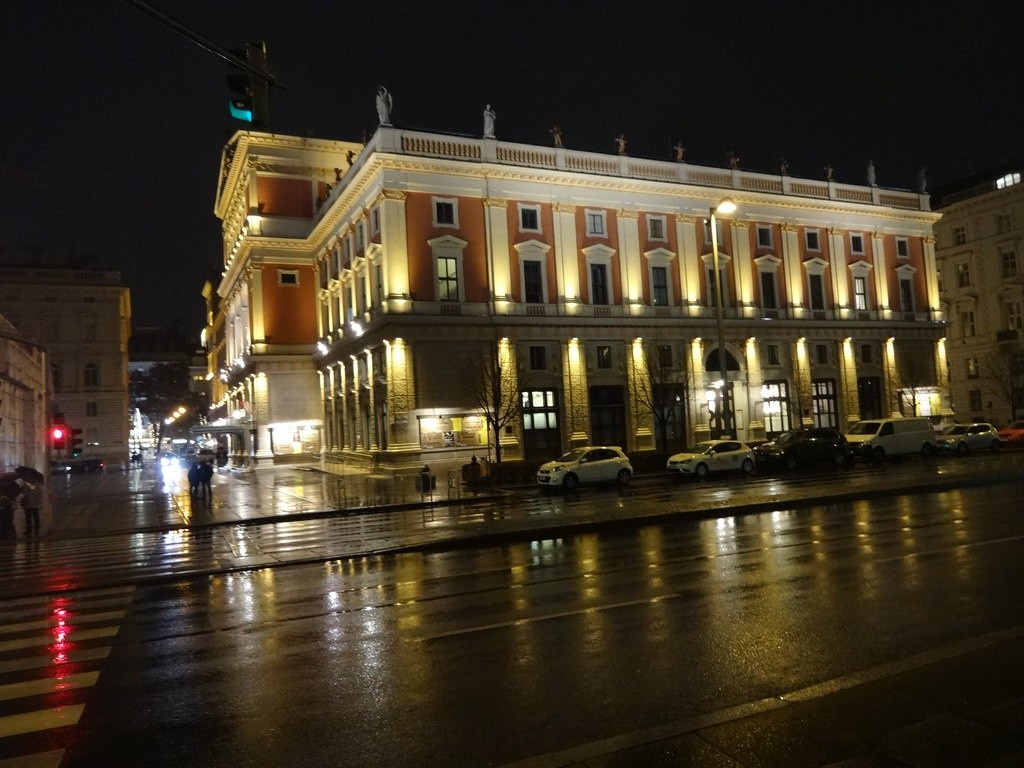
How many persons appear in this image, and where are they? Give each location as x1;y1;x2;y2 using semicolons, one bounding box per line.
727;150;739;167
824;164;834;179
483;104;497;138
346;150;356;167
324;183;332;197
362;129;368;148
673;143;685;161
614;133;628;153
334;168;342;182
779;157;788;174
468;456;491;497
918;168;927;192
375;85;393;125
420;465;432;479
130;453;144;468
15;478;42;536
866;160;875;185
0;495;17;539
315;197;322;210
549;126;562;146
188;463;214;501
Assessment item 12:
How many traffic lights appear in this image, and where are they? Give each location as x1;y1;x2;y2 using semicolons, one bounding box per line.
51;425;66;448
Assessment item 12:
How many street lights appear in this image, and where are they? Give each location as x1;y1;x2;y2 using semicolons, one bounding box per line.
708;195;739;435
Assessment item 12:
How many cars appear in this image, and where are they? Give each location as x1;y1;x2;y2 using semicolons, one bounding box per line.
537;446;634;494
55;459;105;474
997;421;1024;447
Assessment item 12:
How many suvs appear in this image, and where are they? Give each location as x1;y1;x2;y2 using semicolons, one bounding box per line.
754;427;850;471
666;439;756;479
935;423;1000;458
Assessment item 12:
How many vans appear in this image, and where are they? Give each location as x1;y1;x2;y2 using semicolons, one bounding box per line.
844;417;936;463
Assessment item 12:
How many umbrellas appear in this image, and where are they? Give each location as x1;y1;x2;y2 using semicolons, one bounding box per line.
14;465;44;485
0;472;21;499
190;456;211;463
